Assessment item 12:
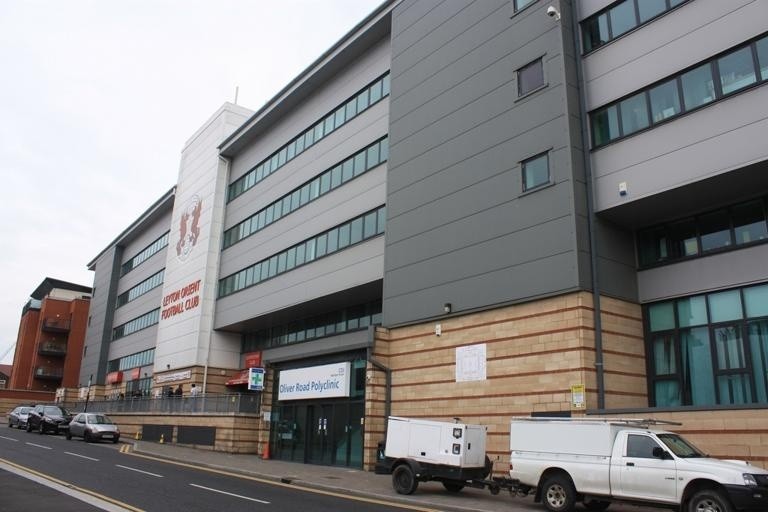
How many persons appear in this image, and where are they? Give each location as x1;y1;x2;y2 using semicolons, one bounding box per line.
102;382;200;413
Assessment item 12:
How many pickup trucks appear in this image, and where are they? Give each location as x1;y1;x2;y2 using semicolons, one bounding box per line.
508;416;768;512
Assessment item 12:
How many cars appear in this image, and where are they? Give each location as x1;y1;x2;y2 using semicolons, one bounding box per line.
8;404;121;442
